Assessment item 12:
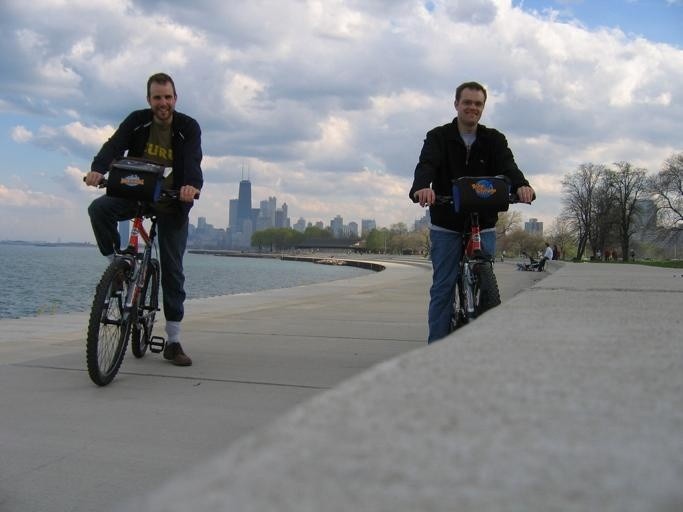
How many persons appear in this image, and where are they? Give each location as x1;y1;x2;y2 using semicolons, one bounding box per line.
408;81;535;342
630;248;635;263
594;249;601;261
612;248;618;263
604;248;609;262
536;243;553;272
85;74;203;366
514;251;532;270
551;243;559;262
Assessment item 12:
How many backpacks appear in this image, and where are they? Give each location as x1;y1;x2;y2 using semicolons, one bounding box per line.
451;175;510;214
106;155;166;205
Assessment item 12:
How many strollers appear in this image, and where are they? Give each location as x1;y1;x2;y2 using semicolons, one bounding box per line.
522;251;539;272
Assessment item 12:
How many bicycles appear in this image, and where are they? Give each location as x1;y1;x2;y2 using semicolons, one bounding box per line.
74;172;204;392
409;182;535;333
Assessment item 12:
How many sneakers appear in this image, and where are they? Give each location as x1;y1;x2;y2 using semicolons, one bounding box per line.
162;341;193;367
